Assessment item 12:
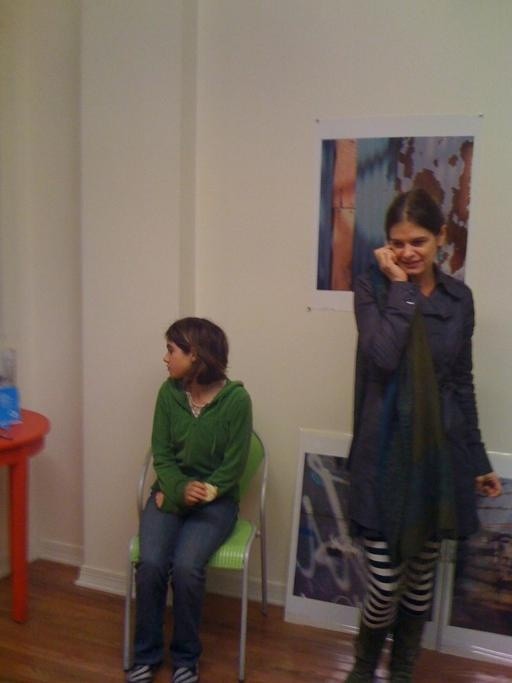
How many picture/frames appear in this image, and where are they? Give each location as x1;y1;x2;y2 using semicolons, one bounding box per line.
433;448;512;670
306;113;485;313
281;427;443;650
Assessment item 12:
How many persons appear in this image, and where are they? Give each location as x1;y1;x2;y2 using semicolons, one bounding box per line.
344;188;502;683
126;318;252;683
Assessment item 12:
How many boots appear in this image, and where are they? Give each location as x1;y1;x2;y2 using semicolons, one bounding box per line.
390;606;427;682
343;618;390;683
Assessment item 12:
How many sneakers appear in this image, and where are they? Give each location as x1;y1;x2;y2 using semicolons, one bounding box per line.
173;661;200;683
126;665;153;683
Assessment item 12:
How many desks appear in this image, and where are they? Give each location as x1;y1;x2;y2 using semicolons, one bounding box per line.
1;408;49;622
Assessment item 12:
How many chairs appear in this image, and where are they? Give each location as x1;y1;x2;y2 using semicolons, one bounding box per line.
122;425;272;683
